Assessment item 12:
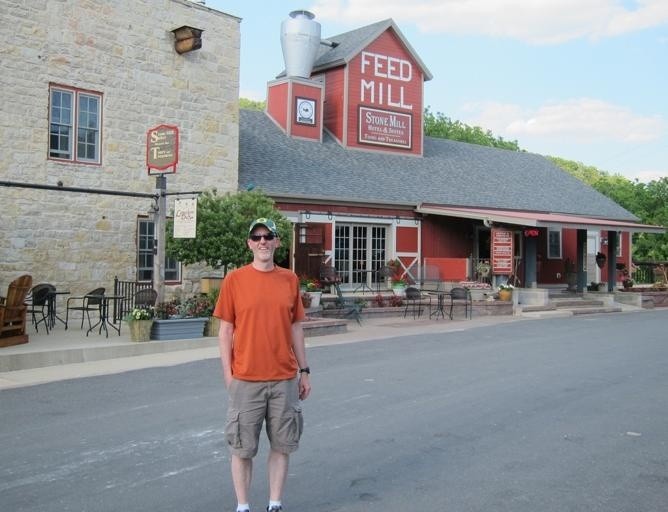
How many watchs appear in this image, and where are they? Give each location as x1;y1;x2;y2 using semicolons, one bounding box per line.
299;367;310;374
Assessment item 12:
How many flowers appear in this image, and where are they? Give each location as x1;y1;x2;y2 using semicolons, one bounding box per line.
306;278;322;291
498;283;513;291
156;291;215;319
376;295;401;306
302;291;314;299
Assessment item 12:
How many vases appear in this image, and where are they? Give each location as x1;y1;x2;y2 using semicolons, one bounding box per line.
302;298;312;308
305;291;322;307
149;318;206;340
499;290;511;301
128;319;154;343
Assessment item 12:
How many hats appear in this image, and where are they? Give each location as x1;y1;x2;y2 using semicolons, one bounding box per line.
248;217;277;236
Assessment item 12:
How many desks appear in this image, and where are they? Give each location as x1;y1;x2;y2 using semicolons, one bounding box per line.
428;292;453;320
351;270;375;294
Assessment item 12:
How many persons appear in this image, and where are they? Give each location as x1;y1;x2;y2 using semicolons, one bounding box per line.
212;218;311;512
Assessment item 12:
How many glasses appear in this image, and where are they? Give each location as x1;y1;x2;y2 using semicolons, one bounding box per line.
248;234;275;242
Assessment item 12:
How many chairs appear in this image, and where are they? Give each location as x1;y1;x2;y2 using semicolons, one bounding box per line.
376;266;395;291
403;286;431;320
448;287;472;321
25;284;158;338
0;273;32;348
320;266;342;296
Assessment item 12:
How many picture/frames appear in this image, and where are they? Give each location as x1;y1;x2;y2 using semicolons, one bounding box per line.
295;96;316;127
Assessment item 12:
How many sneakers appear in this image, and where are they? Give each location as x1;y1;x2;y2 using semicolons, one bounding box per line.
267;503;283;512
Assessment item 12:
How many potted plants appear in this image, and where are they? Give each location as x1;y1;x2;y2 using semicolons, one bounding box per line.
391;280;408;296
